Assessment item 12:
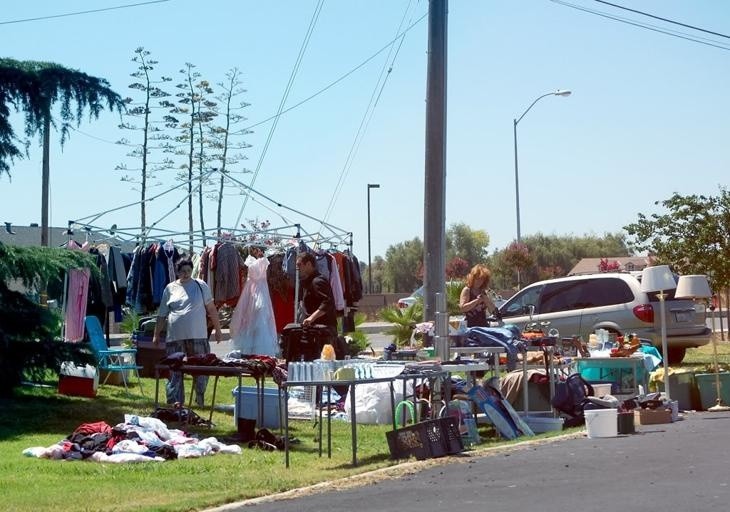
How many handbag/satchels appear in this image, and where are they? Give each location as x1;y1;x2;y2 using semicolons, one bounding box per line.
205;316;215;340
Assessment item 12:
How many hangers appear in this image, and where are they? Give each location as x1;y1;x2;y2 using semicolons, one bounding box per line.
58;232;354;261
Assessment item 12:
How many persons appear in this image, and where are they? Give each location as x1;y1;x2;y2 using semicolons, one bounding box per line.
459;264;503;381
152;257;222;409
295;250;349;404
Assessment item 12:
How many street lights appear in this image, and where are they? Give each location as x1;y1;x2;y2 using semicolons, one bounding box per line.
512;89;573;293
367;183;381;293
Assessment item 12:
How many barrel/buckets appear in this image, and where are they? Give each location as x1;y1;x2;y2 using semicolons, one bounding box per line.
583;409;618;439
583;382;611;398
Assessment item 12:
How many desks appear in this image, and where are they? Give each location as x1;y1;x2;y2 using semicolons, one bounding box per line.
283;347;655;469
155;364;265;431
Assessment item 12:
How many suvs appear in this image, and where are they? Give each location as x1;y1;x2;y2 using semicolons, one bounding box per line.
487;266;713;368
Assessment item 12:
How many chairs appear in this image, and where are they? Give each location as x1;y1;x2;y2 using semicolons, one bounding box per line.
84;316;145;398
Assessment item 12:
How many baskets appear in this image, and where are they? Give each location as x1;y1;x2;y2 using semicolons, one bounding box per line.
385;417;465;459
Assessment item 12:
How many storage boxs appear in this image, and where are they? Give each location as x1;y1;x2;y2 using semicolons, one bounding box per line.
696;373;730;410
232;386;288;432
449;321;468;346
100;356;129;385
658;371;695;410
58;373;96;397
639;407;673;424
